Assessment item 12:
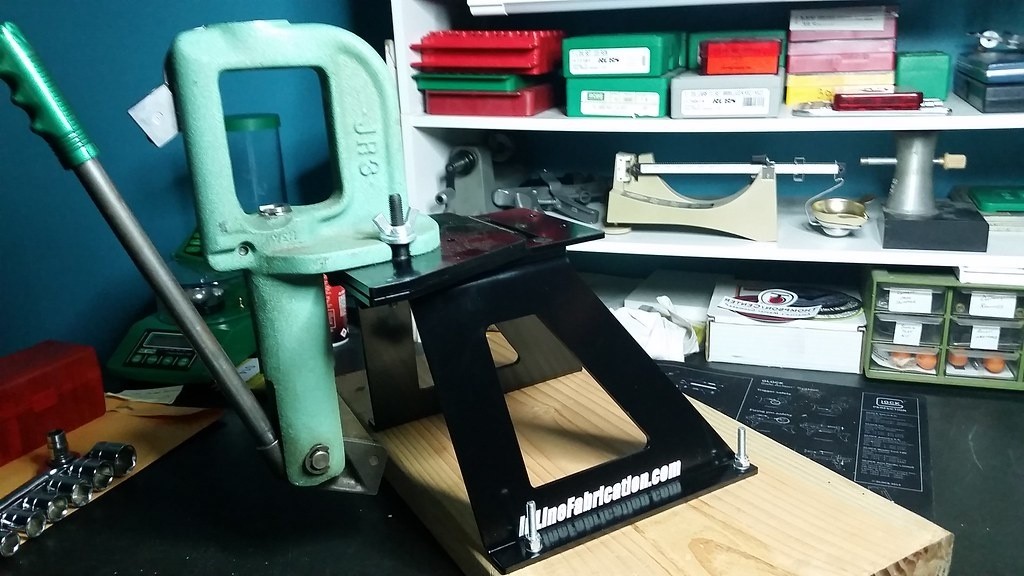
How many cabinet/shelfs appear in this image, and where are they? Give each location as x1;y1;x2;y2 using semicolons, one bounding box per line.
388;0;1024;576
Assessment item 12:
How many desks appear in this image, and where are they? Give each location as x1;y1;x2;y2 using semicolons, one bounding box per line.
0;329;956;576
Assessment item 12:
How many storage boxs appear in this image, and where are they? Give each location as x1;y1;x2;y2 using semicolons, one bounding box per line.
787;39;897;73
895;50;951;101
698;39;783;76
565;72;671;118
788;9;899;41
785;72;894;107
869;344;941;375
687;30;786;67
576;270;641;311
873;314;945;345
562;33;683;79
671;71;786;118
624;267;711;346
704;281;867;374
947;317;1023;349
945;348;1021;381
952;287;1024;321
874;282;947;316
